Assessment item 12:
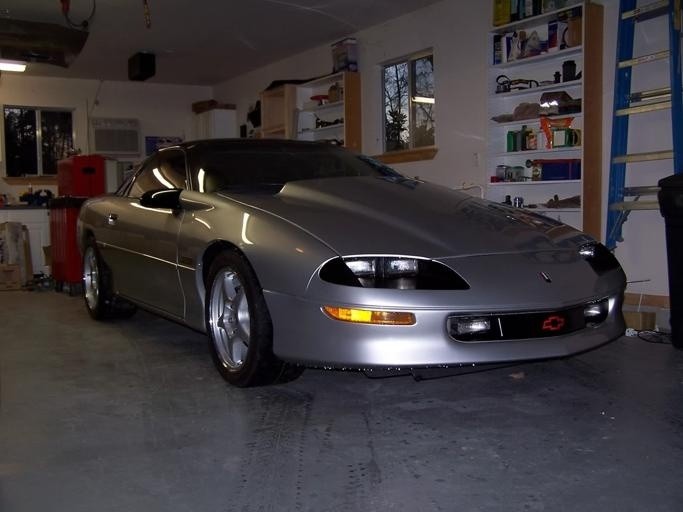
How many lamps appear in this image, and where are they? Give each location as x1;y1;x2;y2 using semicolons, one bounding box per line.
409;91;434;104
0;58;28;72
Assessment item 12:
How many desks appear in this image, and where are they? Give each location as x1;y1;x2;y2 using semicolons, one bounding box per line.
0;193;84;211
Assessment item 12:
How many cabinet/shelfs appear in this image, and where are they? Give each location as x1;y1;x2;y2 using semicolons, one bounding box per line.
259;84;295;142
489;1;603;242
297;73;362;152
177;106;237;146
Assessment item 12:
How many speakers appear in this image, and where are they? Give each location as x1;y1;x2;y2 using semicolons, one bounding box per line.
127;53;155;82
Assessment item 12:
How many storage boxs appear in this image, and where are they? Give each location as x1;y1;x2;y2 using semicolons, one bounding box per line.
622;304;655;333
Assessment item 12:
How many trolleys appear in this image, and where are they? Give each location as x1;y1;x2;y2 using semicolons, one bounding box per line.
44;194;89;297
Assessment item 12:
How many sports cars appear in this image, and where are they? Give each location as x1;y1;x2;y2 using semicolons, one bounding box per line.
75;136;629;390
